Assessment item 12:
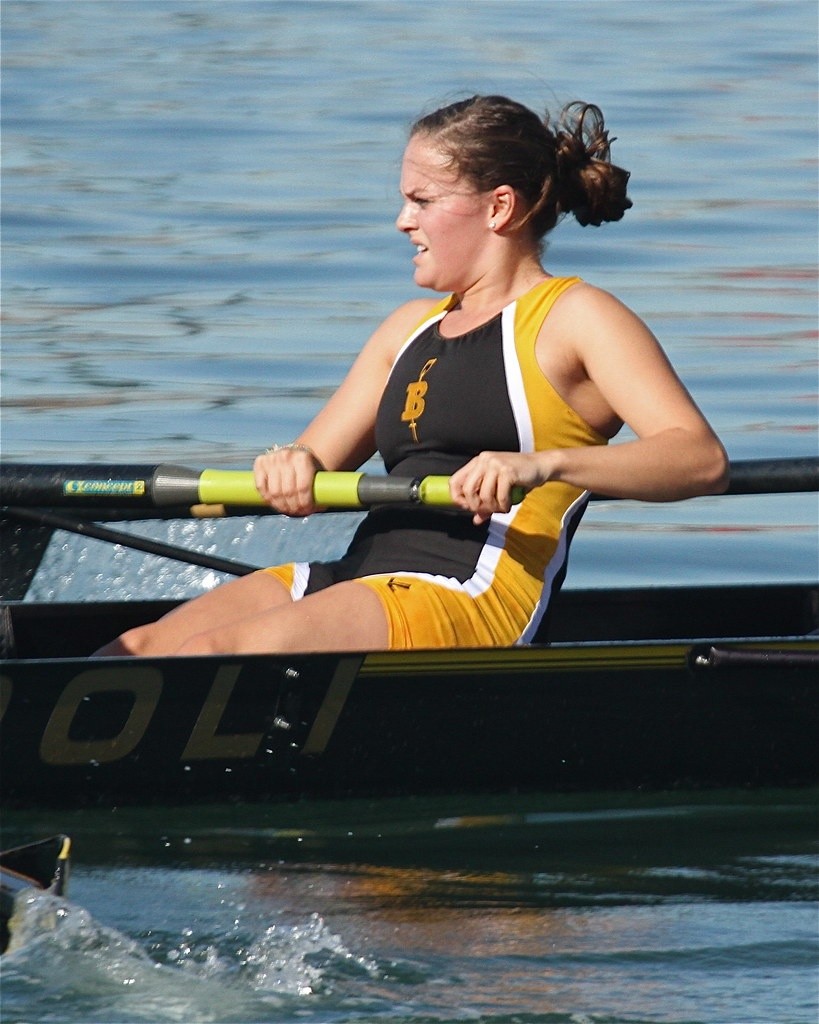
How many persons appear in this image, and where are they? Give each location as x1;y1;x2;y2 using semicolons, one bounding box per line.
94;94;727;658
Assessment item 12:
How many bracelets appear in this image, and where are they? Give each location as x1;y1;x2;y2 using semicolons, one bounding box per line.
265;443;309;453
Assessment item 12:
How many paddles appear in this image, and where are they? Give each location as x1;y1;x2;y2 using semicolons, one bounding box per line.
97;454;818;524
0;461;529;511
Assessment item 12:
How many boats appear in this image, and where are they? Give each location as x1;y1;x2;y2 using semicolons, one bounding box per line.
1;584;818;811
